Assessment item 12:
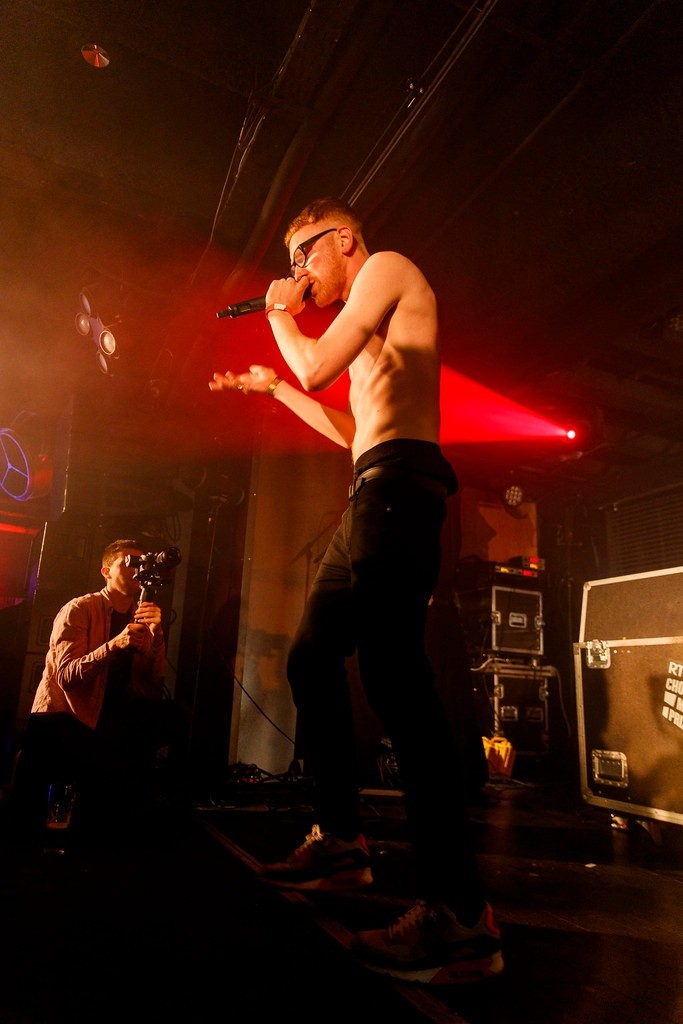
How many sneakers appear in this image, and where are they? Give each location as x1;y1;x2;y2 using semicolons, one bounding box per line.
252;824;373;889
350;897;503;985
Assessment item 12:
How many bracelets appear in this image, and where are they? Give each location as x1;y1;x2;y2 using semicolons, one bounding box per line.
264;303;296;320
267;375;285;398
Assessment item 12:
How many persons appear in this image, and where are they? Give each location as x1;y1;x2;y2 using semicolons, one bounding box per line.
30;537;168;756
206;192;505;983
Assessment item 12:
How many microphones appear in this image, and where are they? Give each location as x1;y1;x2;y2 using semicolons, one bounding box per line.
216;286;311;319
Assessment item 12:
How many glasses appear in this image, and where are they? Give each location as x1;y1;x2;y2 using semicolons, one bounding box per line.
289;229;357;278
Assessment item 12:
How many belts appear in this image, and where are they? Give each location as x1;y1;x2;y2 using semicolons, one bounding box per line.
348;467;448;501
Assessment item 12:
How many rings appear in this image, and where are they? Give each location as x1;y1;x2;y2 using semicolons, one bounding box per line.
235;384;244;391
